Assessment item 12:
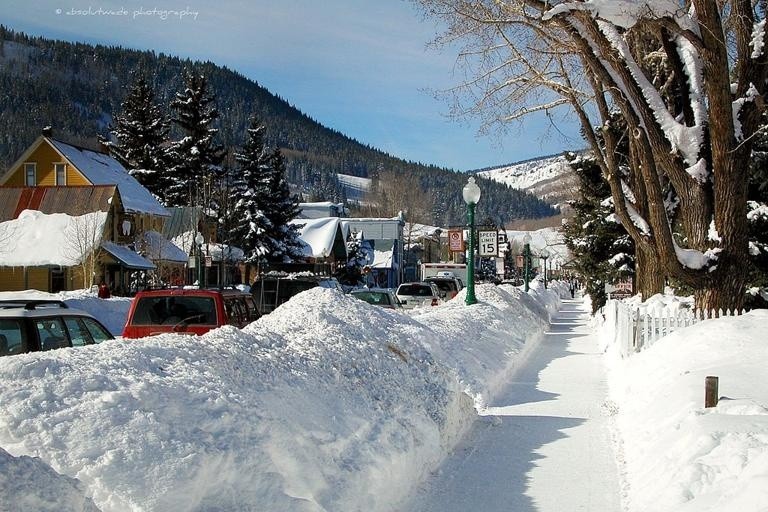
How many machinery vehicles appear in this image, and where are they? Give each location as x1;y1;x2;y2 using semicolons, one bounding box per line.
249;262;347;319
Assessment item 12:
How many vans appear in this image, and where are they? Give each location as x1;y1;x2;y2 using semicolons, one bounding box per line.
424;275;467;303
396;280;447;310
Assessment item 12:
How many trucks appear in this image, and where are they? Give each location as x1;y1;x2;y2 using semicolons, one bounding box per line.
421;263;480;290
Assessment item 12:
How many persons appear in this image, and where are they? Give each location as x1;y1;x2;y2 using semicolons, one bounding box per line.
568;282;574;298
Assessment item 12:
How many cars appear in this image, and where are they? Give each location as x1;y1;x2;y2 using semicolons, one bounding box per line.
348;288;407;309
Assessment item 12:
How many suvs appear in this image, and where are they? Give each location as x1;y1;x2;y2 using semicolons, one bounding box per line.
0;300;116;359
121;284;262;339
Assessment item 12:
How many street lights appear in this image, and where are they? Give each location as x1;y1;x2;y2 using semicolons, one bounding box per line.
548;256;570;287
462;177;481;307
523;232;532;292
541;249;550;289
416;259;421;282
195;232;204;288
